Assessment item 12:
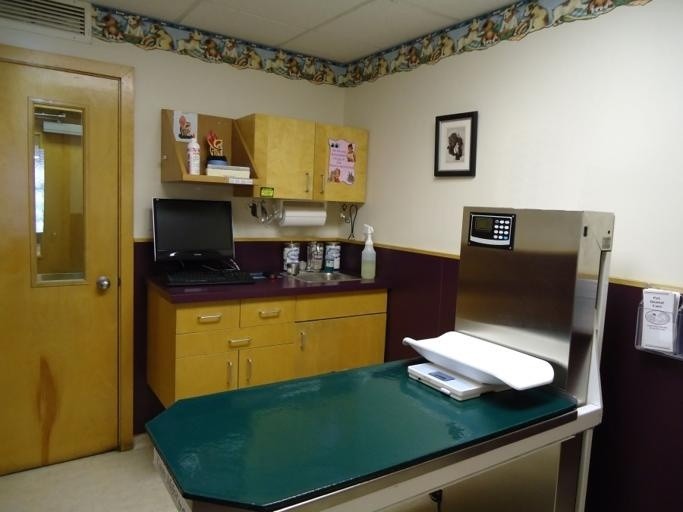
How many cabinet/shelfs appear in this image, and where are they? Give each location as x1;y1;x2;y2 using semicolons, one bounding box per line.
233;112;369;204
161;108;262;186
144;271;412;410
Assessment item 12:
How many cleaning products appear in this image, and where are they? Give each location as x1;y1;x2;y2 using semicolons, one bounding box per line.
361;224;376;279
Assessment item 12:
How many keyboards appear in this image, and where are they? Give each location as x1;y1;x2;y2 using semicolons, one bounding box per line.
162;270;256;285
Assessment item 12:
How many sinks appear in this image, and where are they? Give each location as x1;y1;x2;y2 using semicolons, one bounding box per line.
280;271;362;283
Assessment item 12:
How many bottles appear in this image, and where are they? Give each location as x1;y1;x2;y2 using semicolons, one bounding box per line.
186;133;201;176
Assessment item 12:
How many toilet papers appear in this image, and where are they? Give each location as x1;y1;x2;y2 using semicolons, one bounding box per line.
279;206;326;226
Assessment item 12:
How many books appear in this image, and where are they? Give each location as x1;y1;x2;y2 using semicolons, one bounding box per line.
205;163;250;179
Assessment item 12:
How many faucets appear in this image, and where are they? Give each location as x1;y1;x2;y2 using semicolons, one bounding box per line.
306;244;321;267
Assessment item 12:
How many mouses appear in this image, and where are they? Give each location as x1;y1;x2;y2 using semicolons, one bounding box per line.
262;272;276;279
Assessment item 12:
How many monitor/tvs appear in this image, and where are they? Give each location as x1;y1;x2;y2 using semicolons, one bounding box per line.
152;196;236;269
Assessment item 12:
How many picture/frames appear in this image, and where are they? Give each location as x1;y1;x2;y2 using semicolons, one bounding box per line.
434;110;478;176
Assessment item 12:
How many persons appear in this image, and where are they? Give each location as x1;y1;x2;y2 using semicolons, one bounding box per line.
330;167;341;183
347;143;356;161
